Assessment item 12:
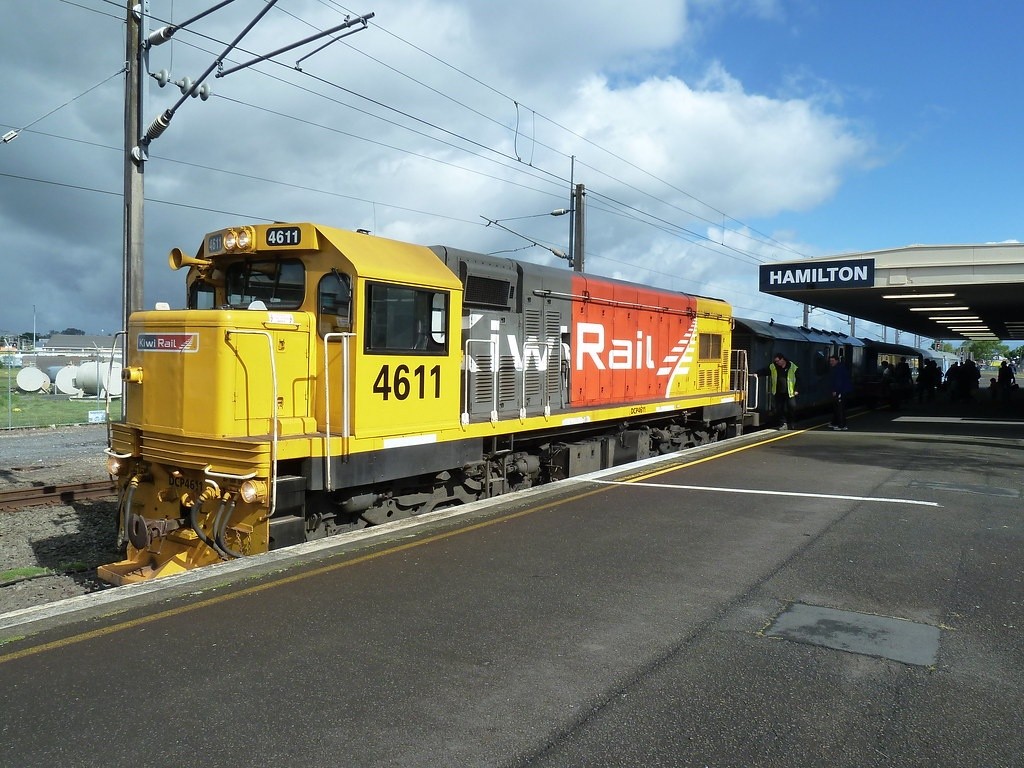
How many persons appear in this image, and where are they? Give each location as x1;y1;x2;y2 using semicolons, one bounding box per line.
872;351;1019;412
828;357;850;431
754;353;801;430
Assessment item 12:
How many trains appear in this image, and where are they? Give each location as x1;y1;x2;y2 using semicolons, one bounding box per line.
95;223;960;585
